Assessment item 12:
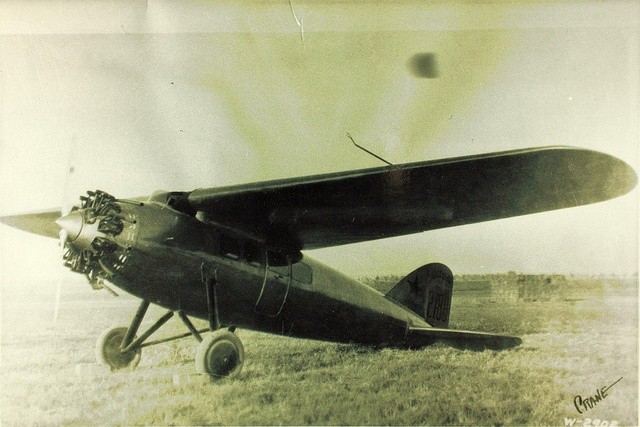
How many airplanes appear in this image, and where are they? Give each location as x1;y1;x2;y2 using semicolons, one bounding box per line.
0;145;637;383
